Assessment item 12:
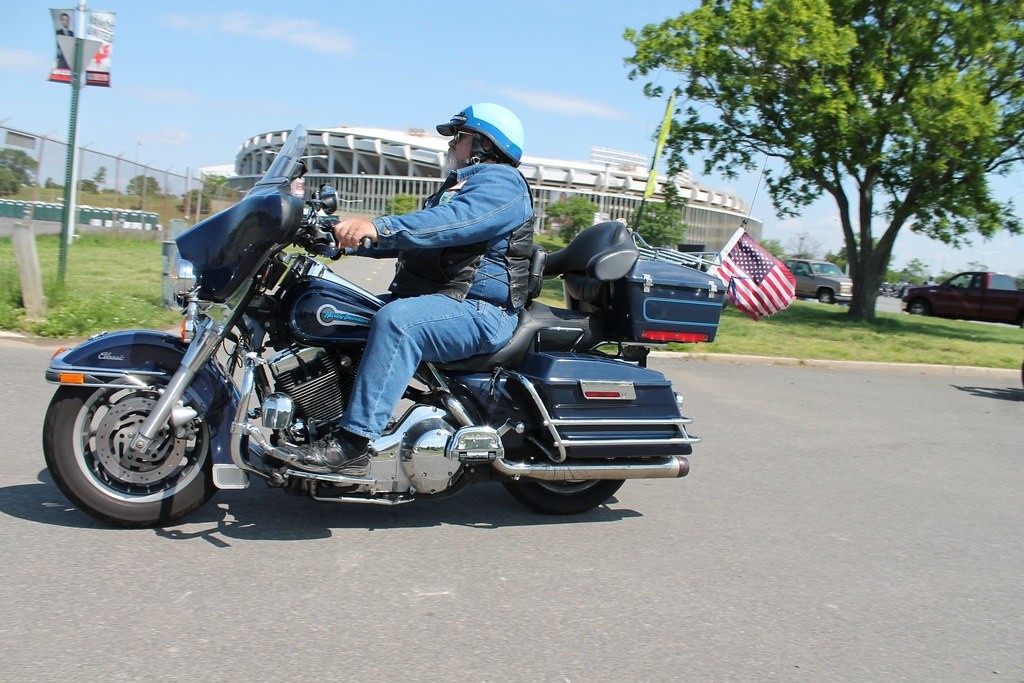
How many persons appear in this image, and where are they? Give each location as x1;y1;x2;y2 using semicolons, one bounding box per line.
56;13;74;69
883;278;913;298
924;276;934;285
271;104;535;478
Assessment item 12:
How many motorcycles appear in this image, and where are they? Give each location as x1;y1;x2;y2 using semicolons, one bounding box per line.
43;126;724;527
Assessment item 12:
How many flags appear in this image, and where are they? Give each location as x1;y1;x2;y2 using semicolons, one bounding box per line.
642;97;675;197
706;227;797;321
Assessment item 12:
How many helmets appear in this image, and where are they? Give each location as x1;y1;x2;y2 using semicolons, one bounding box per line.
437;102;525;166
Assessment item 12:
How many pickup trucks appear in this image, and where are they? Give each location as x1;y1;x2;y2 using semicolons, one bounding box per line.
902;271;1024;329
781;259;854;304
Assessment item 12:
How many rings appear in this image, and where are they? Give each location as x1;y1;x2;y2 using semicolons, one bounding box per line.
347;232;353;235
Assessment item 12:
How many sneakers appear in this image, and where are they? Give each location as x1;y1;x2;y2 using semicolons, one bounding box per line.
273;429;369;478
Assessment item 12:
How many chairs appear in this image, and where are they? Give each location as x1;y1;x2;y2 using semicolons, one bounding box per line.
796;265;806;275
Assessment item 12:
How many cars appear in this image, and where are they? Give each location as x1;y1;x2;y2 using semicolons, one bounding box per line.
879;282;917;298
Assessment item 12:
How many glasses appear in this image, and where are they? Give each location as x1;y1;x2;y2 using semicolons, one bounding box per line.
454;130;473;142
